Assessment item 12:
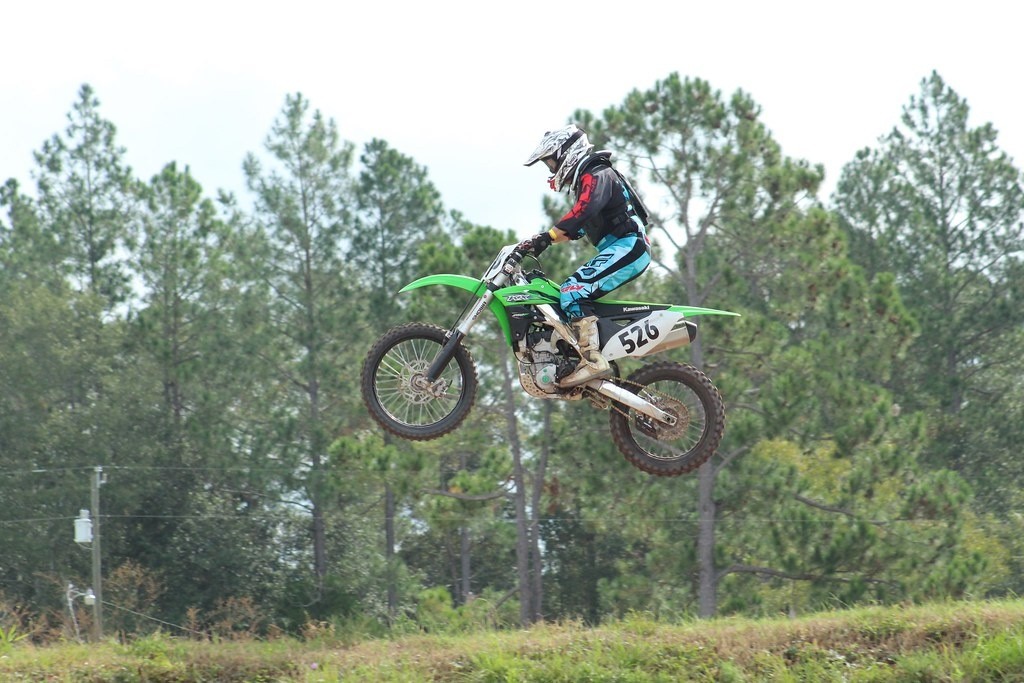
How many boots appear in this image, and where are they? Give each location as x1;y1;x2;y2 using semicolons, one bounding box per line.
559;316;613;388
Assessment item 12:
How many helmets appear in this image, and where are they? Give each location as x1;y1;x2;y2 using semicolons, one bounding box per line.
523;124;595;197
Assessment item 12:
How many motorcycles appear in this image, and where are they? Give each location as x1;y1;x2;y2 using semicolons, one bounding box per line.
362;238;745;477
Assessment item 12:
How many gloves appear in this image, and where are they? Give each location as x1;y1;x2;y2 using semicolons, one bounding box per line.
521;232;555;257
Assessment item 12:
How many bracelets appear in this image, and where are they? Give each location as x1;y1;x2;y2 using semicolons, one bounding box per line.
548;229;558;240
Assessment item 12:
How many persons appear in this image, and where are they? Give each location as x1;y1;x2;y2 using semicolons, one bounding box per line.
522;124;653;387
